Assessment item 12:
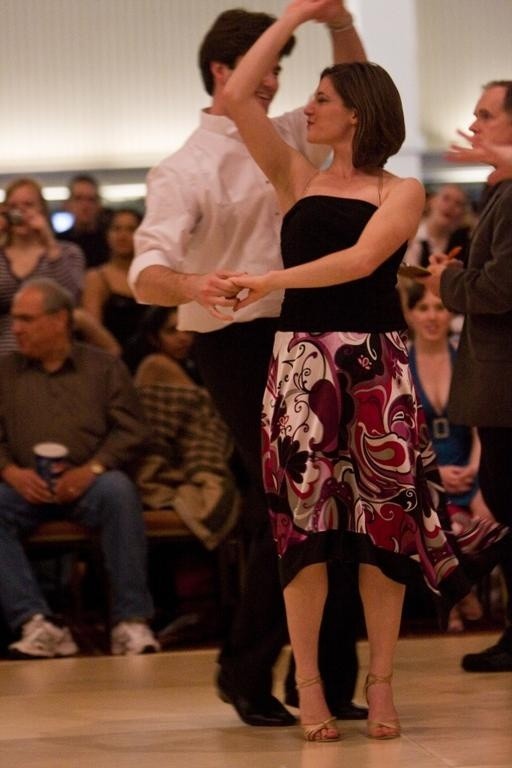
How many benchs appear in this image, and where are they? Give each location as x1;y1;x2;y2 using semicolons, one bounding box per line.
16;504;512;641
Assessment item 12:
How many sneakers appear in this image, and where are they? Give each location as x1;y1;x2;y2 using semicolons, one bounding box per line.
9;613;77;657
111;622;159;655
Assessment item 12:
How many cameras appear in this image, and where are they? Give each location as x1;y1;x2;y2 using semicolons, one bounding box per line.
6;208;24;227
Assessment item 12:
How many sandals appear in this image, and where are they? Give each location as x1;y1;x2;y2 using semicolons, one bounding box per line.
297;683;339;742
366;676;400;737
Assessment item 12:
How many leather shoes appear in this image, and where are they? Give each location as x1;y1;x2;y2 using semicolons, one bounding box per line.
216;673;368;725
463;627;512;672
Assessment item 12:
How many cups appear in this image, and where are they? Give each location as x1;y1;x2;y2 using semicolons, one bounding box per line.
33;442;67;497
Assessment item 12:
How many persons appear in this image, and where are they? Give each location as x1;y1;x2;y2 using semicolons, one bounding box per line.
135;142;483;653
443;126;512;172
0;280;162;656
127;1;365;733
58;170;112;272
84;460;108;479
215;3;425;743
324;13;354;33
0;178;84;368
422;79;512;675
79;206;164;366
